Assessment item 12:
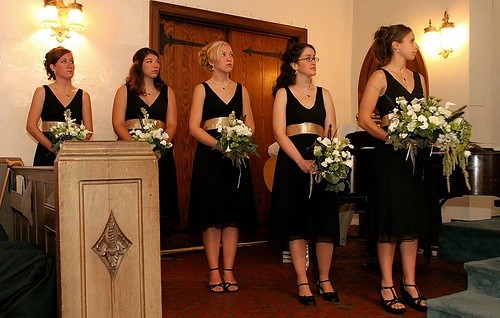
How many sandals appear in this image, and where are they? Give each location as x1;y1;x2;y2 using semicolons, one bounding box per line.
207;268;225;294
221;268;240;292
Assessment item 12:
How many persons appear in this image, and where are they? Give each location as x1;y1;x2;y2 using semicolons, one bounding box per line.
270;43;341;306
188;41;256;295
0;240;57;318
112;48;181;233
358;25;442;314
26;46;93;166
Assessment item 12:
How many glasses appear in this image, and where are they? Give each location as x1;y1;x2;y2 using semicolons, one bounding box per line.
293;57;319;62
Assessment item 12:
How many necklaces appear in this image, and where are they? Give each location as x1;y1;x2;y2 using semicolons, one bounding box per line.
211;78;225;90
403;78;406;82
66;95;69;98
148;93;150;95
294;85;312;97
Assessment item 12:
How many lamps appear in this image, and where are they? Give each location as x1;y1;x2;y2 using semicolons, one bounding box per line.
41;0;86;43
423;11;454;58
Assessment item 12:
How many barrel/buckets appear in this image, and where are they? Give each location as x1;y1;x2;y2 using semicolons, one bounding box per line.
449;148;500;198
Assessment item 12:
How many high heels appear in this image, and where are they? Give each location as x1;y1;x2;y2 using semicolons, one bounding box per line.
399;280;427;312
297;281;316;306
377;280;407;315
316;277;340;303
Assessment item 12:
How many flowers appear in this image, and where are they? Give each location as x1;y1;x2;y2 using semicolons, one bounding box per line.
129;107;172;154
305;124;355;193
365;78;494;193
212;110;260;166
41;108;94;158
